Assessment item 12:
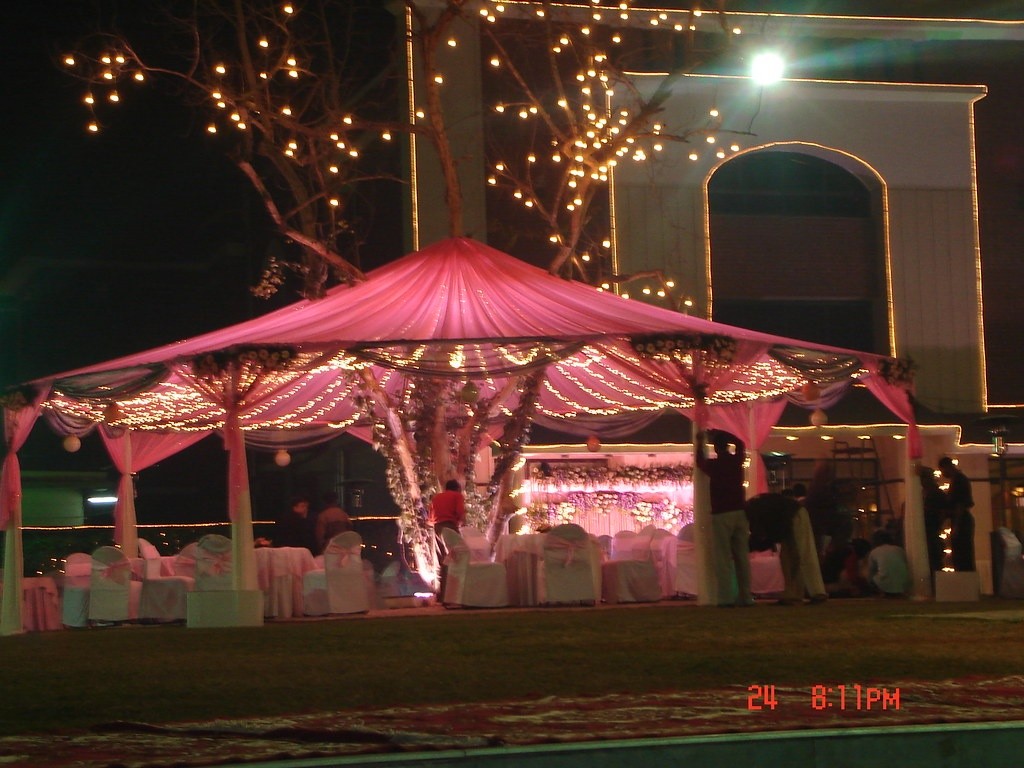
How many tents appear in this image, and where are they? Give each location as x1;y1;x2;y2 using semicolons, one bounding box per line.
0;237;932;637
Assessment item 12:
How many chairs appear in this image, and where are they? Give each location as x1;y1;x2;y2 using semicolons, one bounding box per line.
0;522;781;632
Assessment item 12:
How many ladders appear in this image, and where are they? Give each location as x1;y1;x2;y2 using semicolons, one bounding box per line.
832;436;895;538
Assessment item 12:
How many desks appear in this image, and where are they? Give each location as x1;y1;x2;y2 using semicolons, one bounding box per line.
498;531;546;605
253;548;321;620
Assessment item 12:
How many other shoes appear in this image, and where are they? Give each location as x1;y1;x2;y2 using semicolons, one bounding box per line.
739;601;758;607
717;603;735;608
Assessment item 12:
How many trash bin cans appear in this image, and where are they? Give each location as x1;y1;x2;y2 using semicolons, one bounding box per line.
990;526;1024;596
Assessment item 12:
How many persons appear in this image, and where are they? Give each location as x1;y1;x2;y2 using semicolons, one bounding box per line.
272;490;354;558
428;479;466;609
695;430;975;609
